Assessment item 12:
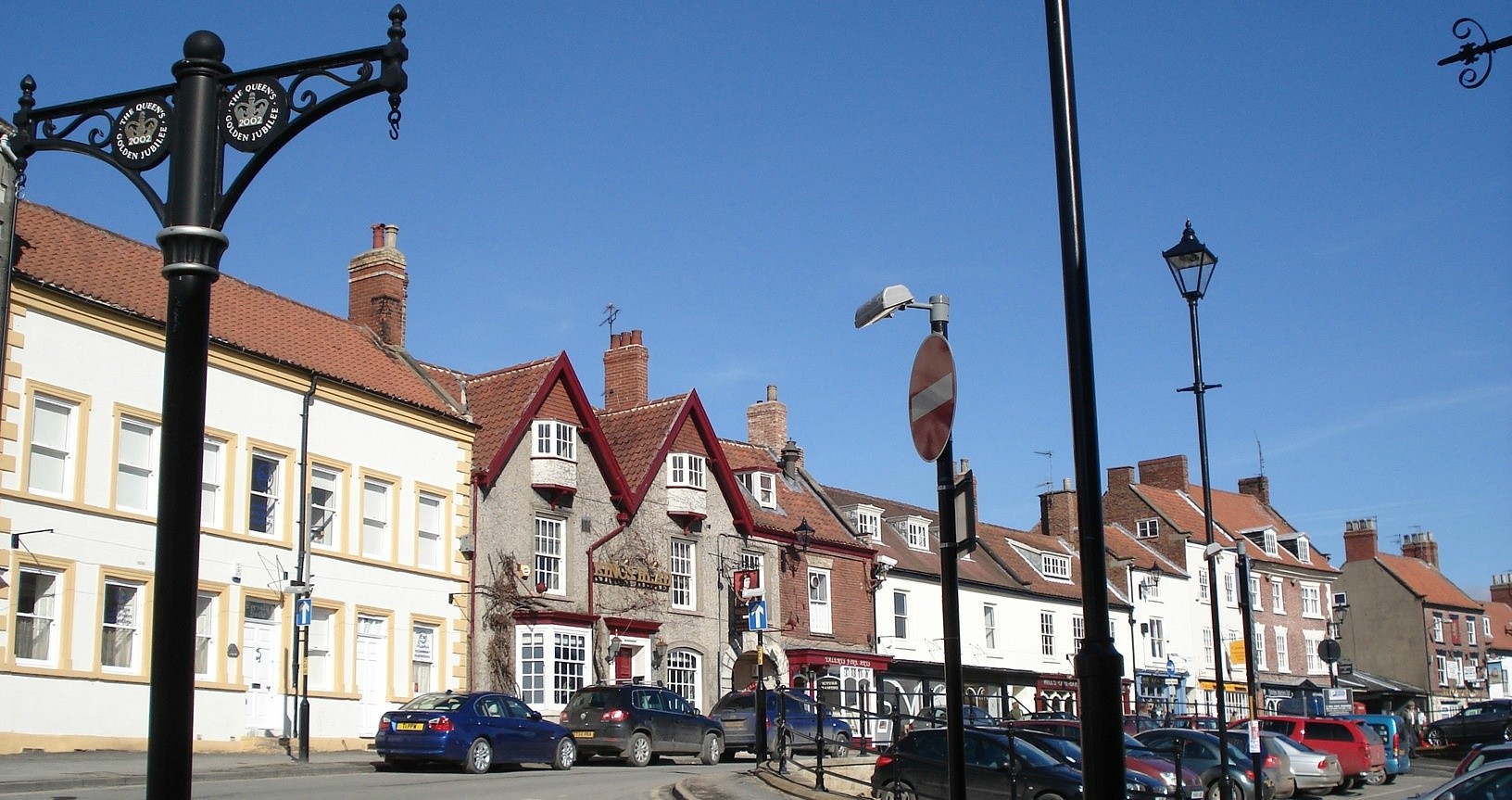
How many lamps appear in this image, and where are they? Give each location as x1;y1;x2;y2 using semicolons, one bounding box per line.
605;629;622;666
779;516;816;573
651;636;669;670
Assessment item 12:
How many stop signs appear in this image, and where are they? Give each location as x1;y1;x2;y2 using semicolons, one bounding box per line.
906;329;958;462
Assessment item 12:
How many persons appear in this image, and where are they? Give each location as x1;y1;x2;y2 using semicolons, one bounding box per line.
1229;711;1237;722
734;574;758;607
1401;701;1420;758
1010;702;1023;720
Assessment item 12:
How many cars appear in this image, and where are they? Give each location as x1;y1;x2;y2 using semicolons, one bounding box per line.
871;725;1168;800
555;684;728;765
706;687;855;760
1017;699;1512;800
370;690;580;772
905;703;1000;727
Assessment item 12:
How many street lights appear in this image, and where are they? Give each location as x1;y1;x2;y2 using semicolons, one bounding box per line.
849;285;977;800
1161;216;1243;800
